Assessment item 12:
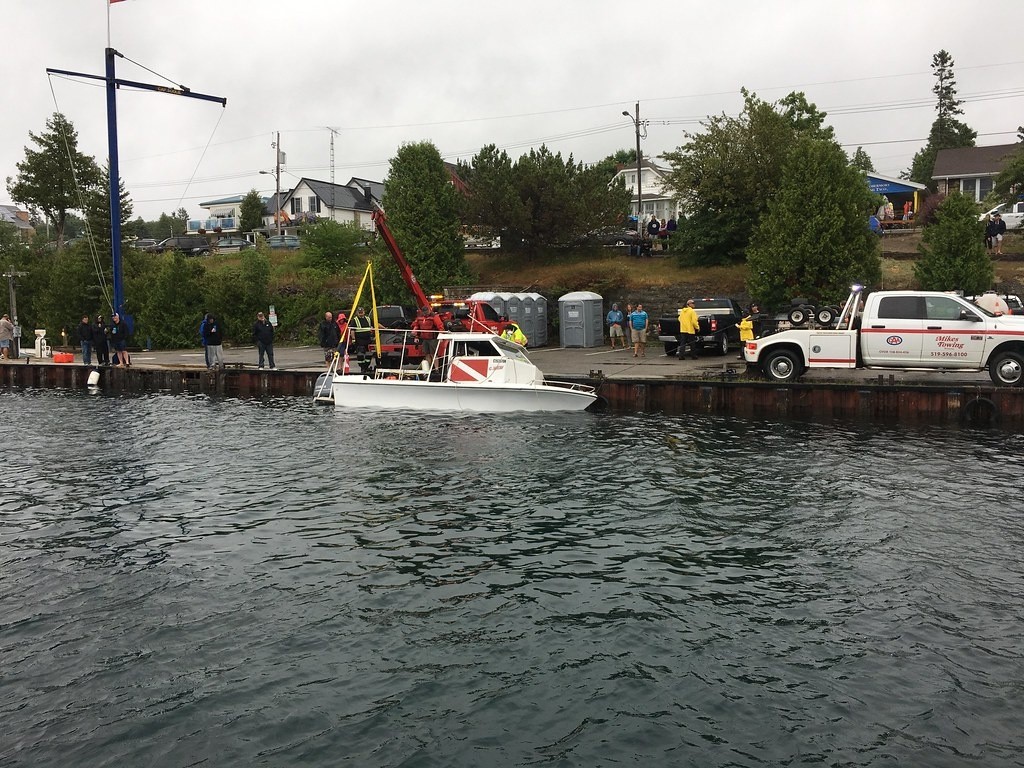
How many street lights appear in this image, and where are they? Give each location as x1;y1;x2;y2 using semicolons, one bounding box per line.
622;103;643;239
258;170;282;235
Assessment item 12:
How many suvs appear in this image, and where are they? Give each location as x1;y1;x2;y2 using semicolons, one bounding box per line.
144;236;212;257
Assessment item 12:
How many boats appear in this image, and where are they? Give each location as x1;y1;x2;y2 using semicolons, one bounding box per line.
313;258;599;414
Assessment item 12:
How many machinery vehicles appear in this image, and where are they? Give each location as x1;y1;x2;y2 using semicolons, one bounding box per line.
368;197;528;364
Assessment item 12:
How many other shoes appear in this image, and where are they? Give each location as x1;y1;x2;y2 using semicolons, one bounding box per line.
622;345;626;348
269;366;278;370
610;346;615;350
363;375;367;380
116;363;123;367
690;355;699;359
434;369;439;373
125;363;130;365
83;363;93;366
633;354;639;357
641;353;645;357
258;366;264;370
679;356;685;360
627;347;632;350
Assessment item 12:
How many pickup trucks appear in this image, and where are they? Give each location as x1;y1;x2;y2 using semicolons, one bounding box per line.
743;290;1024;387
657;298;745;355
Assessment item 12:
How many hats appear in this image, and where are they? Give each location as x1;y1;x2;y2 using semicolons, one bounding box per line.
357;306;364;312
256;311;264;317
686;300;694;304
111;313;119;317
421;306;429;314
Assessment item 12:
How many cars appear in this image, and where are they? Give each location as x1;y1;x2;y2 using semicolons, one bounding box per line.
595;231;652;253
977;202;1024;230
134;238;160;252
190;238;259;255
369;304;406;326
264;235;302;249
960;294;1023;318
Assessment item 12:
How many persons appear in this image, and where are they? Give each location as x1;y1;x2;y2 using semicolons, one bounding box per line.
735;309;756;360
318;312;342;374
252;312;278;371
109;312;130;368
606;303;626;349
621;303;635;349
666;215;677;249
335;313;352;371
869;214;885;238
677;299;701;360
647;216;660;251
200;313;225;368
349;307;386;355
625;217;636;232
78;314;113;367
752;303;762;339
0;314;15;361
840;300;856;329
499;323;529;350
658;218;668;250
982;213;1006;255
677;211;687;224
626;231;653;258
629;303;649;358
411;306;444;374
